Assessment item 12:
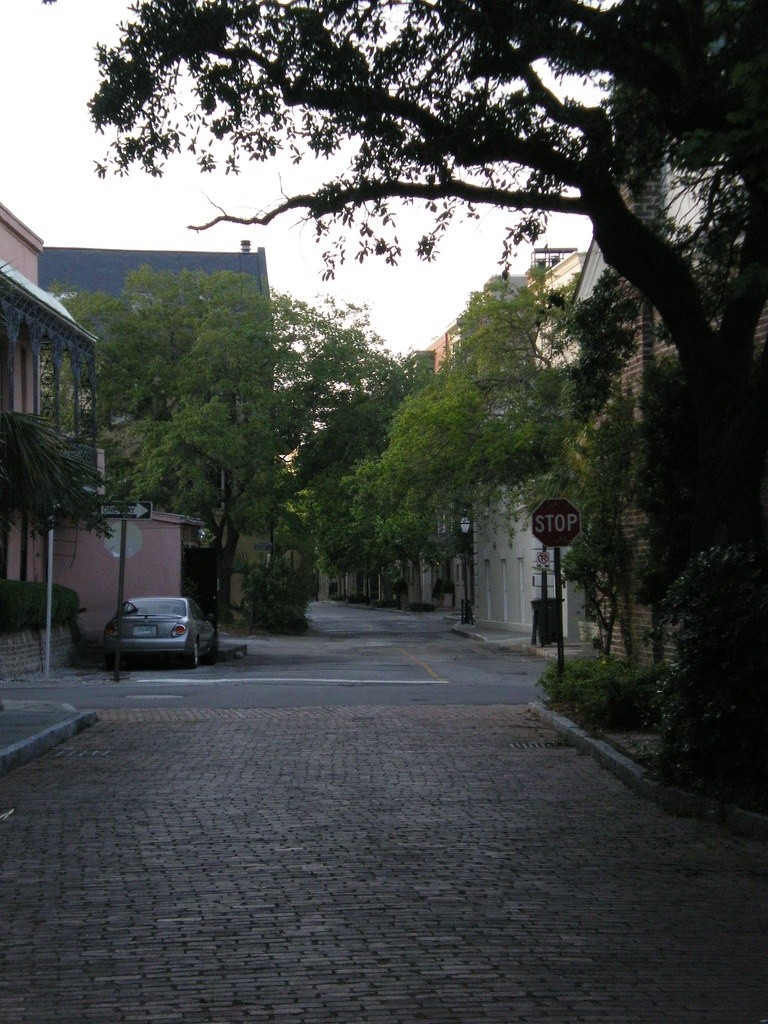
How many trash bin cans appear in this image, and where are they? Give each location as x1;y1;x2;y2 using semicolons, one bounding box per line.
531;597;565;645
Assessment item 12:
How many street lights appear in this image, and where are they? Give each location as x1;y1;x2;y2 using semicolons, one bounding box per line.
460;507;473;626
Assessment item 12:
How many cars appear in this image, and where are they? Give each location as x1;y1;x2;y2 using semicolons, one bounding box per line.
101;595;217;668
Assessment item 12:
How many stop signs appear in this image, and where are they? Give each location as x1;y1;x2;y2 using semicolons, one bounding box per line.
531;497;581;546
